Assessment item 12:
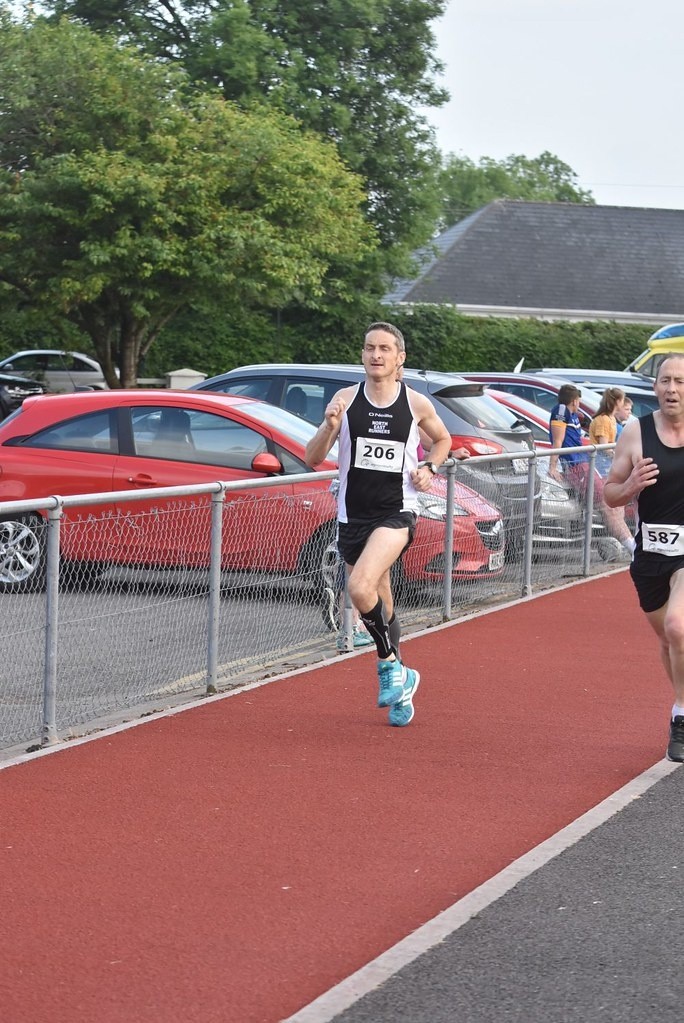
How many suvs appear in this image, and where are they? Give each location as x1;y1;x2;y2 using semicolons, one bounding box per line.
91;363;545;544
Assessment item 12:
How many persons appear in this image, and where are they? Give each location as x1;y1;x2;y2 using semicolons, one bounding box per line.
548;383;637;556
589;387;626;521
321;366;471;648
305;324;452;726
604;353;684;763
615;397;633;444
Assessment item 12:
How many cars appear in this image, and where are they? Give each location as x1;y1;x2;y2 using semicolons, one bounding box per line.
443;367;661;558
0;372;43;417
0;389;511;613
0;348;124;395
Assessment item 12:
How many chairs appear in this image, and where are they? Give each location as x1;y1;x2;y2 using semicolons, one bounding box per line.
285;386;309;416
149;410;199;462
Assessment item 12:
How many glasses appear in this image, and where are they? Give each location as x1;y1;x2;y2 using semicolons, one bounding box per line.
577;389;582;398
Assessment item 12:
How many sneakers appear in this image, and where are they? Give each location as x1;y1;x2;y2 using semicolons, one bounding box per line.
387;665;421;726
376;659;407;708
665;715;684;762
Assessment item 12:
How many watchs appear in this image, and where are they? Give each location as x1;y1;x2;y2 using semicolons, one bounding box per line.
423;462;438;475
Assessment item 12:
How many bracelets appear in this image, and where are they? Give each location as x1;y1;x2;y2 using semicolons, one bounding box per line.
449;450;453;459
